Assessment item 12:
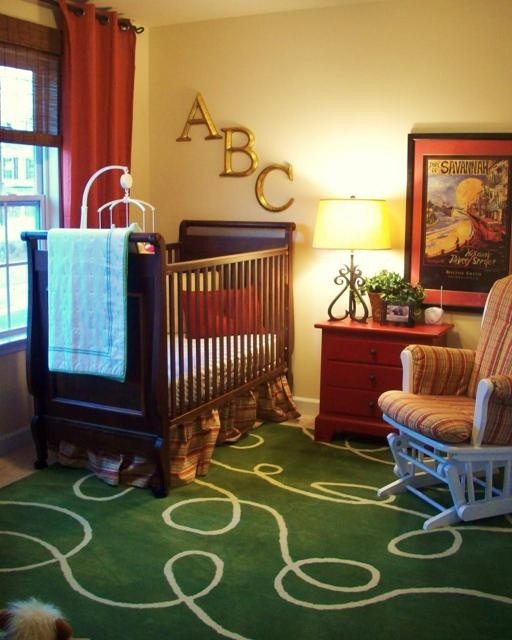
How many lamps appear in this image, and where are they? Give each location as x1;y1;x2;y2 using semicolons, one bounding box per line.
312;195;391;322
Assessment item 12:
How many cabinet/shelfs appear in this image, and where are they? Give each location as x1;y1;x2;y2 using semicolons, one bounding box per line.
314;318;454;444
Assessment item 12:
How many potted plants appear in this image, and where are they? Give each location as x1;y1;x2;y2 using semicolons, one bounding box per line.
361;269;424;323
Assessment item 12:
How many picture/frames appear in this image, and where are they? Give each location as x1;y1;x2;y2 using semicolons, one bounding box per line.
380;300;415;327
403;133;511;313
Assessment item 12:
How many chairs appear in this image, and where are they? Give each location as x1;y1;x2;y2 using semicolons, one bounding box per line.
375;275;512;530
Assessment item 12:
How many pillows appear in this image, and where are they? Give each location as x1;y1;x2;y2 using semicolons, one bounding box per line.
165;271;269;339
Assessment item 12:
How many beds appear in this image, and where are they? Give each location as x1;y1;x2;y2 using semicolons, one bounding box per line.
20;221;296;496
